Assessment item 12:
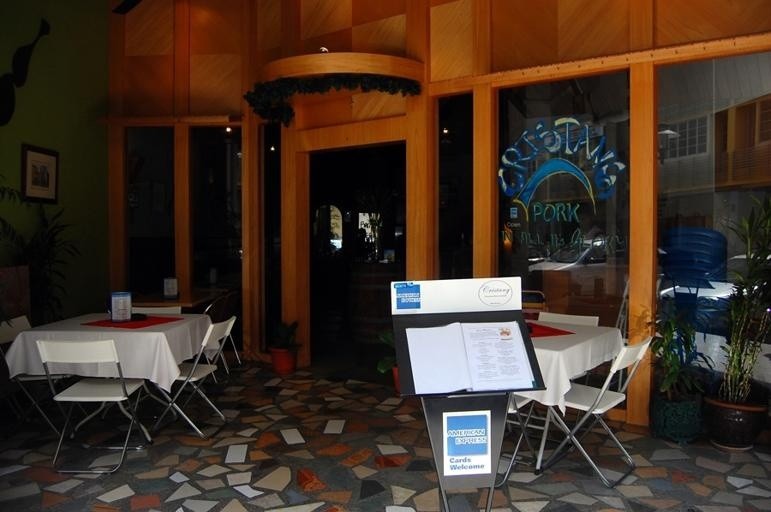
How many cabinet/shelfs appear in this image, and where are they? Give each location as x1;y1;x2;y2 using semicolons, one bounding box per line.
713;94;770;191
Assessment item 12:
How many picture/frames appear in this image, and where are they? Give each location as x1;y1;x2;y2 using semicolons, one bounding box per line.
20;143;61;205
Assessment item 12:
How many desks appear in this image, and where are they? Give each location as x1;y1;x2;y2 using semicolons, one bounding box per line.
3;312;221;449
504;326;623;475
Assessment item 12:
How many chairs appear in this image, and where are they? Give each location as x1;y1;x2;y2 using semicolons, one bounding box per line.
0;317;70;444
150;315;238;438
35;340;147;476
196;293;242;379
540;335;653;490
129;306;183;315
538;310;600;327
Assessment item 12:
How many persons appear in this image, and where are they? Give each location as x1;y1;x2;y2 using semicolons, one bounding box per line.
331;205;343;248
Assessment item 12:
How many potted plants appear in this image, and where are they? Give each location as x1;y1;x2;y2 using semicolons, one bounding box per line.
644;274;718;447
704;194;770;454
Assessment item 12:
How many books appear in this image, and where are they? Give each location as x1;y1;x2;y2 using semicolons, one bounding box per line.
404;320;538;395
